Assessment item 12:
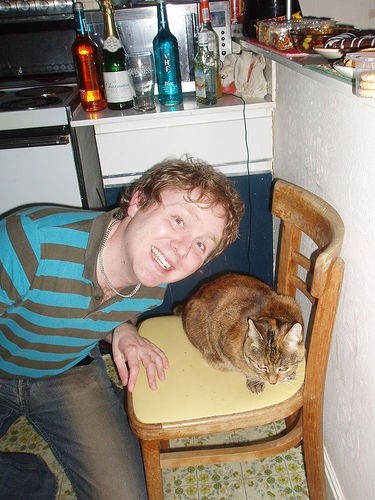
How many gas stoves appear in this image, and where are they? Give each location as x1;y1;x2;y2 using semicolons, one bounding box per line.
0;0;86;134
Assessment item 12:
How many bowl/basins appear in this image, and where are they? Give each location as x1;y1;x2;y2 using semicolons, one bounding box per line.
289;24;360;53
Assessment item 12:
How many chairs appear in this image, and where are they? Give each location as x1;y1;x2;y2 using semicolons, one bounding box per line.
127;179;345;500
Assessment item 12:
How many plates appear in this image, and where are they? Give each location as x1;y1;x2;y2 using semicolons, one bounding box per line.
312;45;356;62
332;59;375;79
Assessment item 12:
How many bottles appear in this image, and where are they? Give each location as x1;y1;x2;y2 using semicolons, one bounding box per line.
71;1;108;112
97;0;137;111
150;0;184;106
192;1;221;105
193;33;219;105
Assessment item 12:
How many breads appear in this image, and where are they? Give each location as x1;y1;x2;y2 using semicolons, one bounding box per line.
357;70;375;98
324;30;375;68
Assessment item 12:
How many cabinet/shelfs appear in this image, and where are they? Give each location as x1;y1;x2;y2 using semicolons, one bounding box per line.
69;108;276;210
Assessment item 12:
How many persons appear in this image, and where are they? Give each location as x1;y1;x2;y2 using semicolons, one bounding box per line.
0;155;243;500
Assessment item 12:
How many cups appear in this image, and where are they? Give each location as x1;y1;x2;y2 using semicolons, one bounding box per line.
356;54;375;98
123;52;157;112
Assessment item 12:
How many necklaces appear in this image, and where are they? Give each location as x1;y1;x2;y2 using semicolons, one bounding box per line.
99;219;141;297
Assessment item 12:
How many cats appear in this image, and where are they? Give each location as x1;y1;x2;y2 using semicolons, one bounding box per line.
174;273;306;394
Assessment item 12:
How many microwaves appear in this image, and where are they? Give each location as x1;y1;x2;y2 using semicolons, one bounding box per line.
79;0;233;99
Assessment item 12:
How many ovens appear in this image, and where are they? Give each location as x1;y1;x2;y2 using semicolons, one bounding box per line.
0;128;104;237
